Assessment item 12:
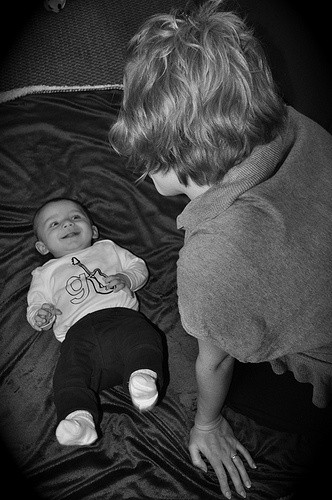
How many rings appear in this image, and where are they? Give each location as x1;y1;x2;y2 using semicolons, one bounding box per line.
231;454;239;460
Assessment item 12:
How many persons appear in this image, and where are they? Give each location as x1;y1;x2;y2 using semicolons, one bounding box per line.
27;197;166;446
106;0;332;500
